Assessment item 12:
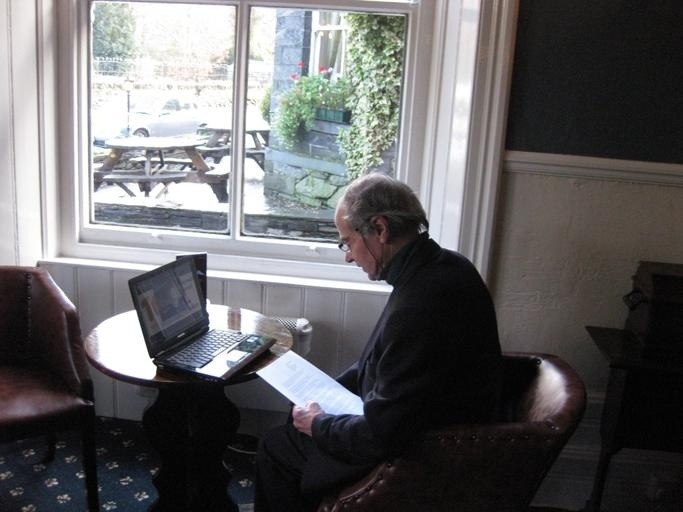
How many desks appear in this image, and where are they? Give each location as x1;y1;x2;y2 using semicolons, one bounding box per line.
586;323;674;510
93;138;213;199
197;124;268;171
82;301;292;511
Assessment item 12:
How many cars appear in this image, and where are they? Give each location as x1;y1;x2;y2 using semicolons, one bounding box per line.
121;97;209;138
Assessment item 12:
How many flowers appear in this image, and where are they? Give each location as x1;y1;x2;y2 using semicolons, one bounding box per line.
275;62;350;146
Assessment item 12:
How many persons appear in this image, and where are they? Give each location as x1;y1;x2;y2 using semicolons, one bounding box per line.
254;173;504;512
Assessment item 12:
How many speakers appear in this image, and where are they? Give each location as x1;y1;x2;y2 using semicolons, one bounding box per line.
175;253;207;307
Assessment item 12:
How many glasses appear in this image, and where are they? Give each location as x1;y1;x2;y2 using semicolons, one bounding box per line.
338;213;387;253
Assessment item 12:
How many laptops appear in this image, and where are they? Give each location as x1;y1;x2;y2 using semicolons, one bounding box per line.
127;255;277;385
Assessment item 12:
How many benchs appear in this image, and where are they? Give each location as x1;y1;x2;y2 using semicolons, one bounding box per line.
92;141;266;205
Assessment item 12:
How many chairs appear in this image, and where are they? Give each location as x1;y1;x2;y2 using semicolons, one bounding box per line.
316;350;586;512
0;266;100;511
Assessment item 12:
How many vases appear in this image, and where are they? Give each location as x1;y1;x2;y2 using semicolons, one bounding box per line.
310;104;351;125
